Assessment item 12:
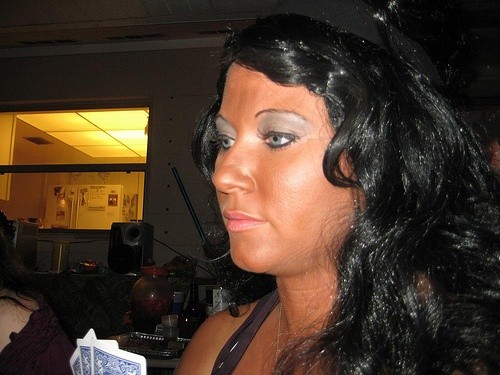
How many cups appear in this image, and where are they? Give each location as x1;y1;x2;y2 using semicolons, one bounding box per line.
163;315;178;338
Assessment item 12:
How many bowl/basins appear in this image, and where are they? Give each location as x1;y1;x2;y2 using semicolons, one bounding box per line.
27;216;39;222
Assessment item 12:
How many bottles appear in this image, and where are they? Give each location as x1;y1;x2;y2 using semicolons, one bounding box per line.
184;279;205;340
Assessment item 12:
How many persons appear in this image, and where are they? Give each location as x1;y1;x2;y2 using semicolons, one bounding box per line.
0;213;77;375
172;1;500;375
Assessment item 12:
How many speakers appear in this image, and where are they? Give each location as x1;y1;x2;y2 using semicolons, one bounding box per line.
108;222;154;275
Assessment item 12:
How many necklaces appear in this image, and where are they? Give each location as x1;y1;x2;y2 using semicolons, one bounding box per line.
276;299;283;363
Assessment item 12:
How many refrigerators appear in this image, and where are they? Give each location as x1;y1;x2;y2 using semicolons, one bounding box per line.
45;185;122;230
